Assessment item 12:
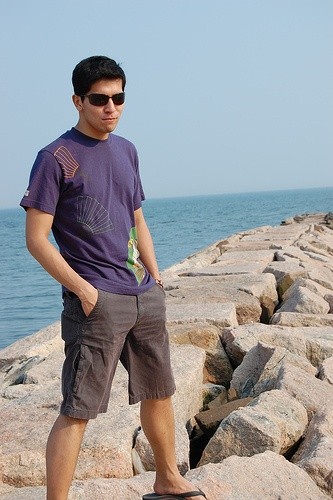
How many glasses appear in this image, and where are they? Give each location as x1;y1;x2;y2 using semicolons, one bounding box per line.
80;92;125;106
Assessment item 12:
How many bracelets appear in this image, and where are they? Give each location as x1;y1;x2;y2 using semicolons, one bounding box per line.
154;278;164;288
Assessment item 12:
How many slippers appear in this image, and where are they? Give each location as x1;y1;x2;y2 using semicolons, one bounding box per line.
142;487;208;500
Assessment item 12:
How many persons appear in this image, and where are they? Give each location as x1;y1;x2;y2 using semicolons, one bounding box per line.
19;55;208;500
